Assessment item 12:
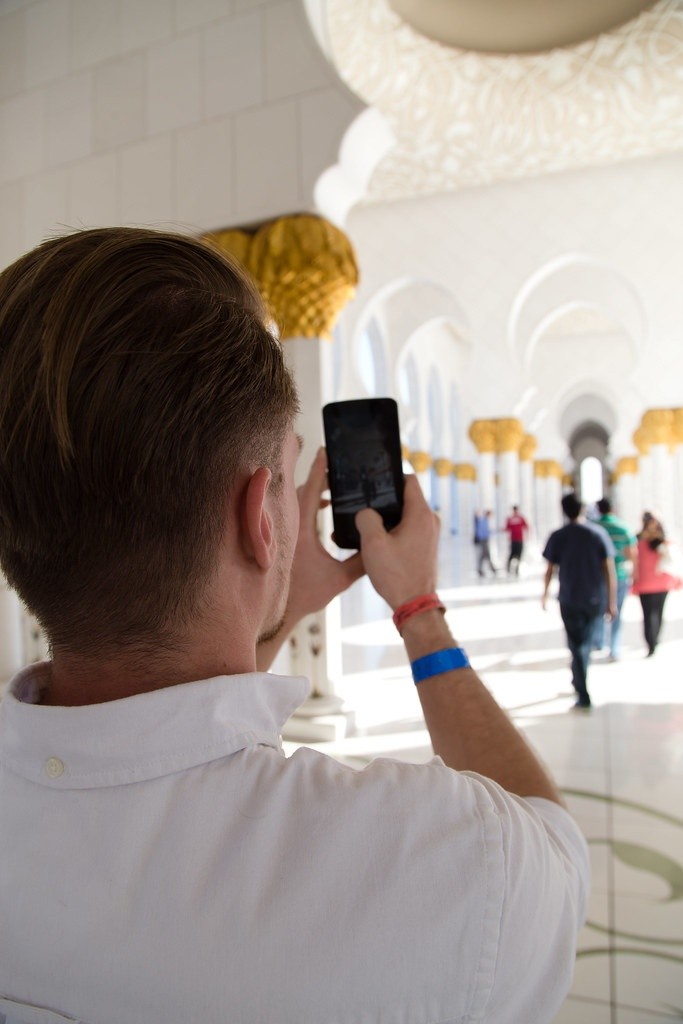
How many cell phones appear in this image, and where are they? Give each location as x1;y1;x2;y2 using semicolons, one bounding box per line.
322;397;405;549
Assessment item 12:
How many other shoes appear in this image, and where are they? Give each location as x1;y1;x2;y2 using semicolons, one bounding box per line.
574;698;591;707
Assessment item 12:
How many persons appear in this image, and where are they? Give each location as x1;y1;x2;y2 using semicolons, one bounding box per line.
577;502;619;674
0;225;593;1024
589;497;631;659
540;494;607;708
477;510;496;576
623;512;683;657
505;505;528;572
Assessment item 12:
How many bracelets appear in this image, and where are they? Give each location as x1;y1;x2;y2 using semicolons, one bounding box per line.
411;647;470;685
393;594;447;635
